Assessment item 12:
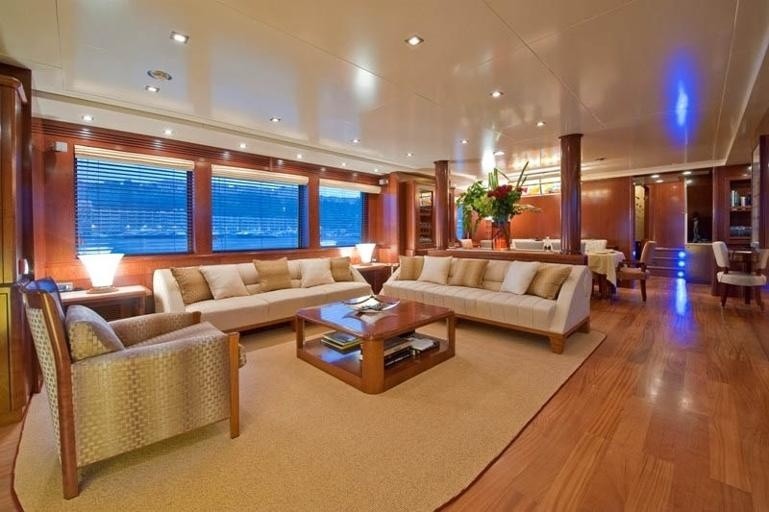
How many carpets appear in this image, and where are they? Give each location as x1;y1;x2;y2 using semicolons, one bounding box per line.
10;316;607;512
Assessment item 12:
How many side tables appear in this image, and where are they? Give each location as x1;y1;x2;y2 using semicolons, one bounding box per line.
352;262;399;294
59;284;152;322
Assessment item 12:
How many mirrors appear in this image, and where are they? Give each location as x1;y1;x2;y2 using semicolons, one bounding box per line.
682;170;715;246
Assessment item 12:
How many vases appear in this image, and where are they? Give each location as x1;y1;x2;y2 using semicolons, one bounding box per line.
491;221;511;252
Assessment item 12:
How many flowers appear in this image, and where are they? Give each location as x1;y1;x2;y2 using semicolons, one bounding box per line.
455;161;542;236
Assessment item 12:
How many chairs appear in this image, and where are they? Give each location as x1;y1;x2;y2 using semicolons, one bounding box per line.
551;241;588;252
510;239;544;250
581;240;607;251
459;238;473;249
480;239;492;249
543;239;561;249
712;241;767;308
617;240;657;302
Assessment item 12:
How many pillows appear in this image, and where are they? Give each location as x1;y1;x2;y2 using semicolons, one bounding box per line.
527;262;573;300
298;257;335;288
417;255;453;286
398;255;424;280
252;256;293;294
500;260;540;295
448;257;489;288
170;266;213;305
330;256;354;282
199;265;249;301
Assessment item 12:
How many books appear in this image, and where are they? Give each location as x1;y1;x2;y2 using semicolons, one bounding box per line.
359;337;439;368
320;331;362;351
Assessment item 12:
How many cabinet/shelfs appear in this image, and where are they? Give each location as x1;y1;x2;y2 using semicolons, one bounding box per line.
405;181;455;257
750;134;769;248
1;62;33;428
725;165;756;249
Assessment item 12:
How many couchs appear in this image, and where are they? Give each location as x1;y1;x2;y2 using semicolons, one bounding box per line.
152;256;374;334
16;276;247;500
378;255;592;355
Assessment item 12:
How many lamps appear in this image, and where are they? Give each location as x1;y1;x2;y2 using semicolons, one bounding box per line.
77;253;125;294
355;242;377;266
